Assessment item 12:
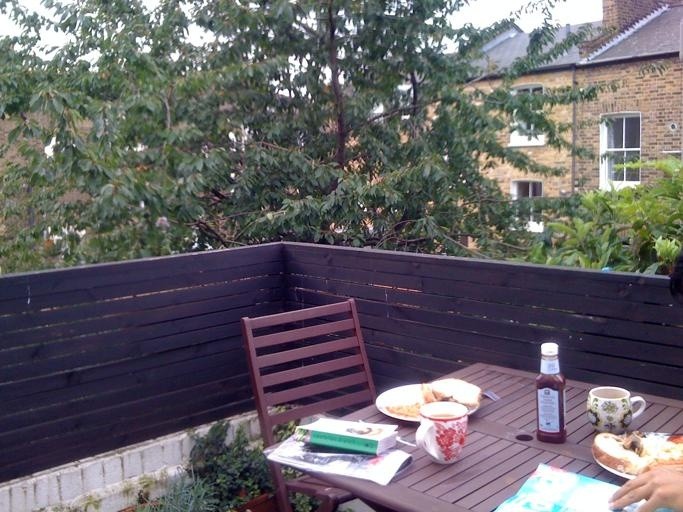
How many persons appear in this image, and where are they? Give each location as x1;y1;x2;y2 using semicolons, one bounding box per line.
608;463;683;512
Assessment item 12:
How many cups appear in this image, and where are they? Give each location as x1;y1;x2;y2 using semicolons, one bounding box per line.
586;385;646;433
415;403;467;464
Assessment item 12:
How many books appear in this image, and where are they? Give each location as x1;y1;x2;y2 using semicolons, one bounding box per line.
292;417;399;456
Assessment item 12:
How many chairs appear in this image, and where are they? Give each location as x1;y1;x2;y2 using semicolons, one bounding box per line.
241;296;391;511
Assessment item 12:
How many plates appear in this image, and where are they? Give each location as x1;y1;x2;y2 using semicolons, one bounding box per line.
375;379;478;426
589;432;682;483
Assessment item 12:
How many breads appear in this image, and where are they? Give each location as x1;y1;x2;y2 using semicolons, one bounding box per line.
593;431;683;477
421;379;483;410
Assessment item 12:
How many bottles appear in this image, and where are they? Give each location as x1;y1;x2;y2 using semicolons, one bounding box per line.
534;342;567;441
